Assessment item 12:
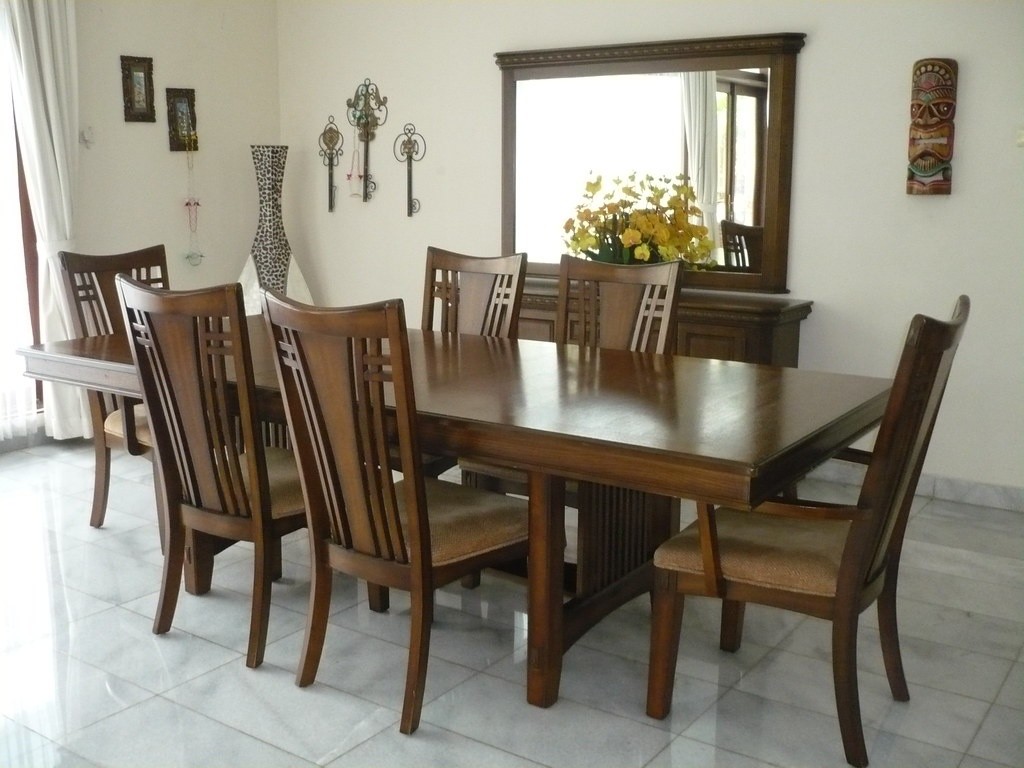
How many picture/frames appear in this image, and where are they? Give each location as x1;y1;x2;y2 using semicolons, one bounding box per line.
165;87;200;151
120;55;157;123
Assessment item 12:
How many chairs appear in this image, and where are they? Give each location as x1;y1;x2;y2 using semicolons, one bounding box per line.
419;246;530;340
553;255;685;355
258;285;531;737
114;269;311;668
56;243;177;559
644;295;974;768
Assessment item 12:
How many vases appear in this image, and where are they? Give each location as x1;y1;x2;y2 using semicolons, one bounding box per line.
234;142;316;317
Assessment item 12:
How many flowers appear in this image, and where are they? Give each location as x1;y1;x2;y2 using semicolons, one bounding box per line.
561;166;724;272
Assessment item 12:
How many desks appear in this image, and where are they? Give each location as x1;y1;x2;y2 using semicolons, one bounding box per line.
505;271;816;373
25;311;897;708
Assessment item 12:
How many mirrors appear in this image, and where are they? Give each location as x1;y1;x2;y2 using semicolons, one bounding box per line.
493;29;809;291
